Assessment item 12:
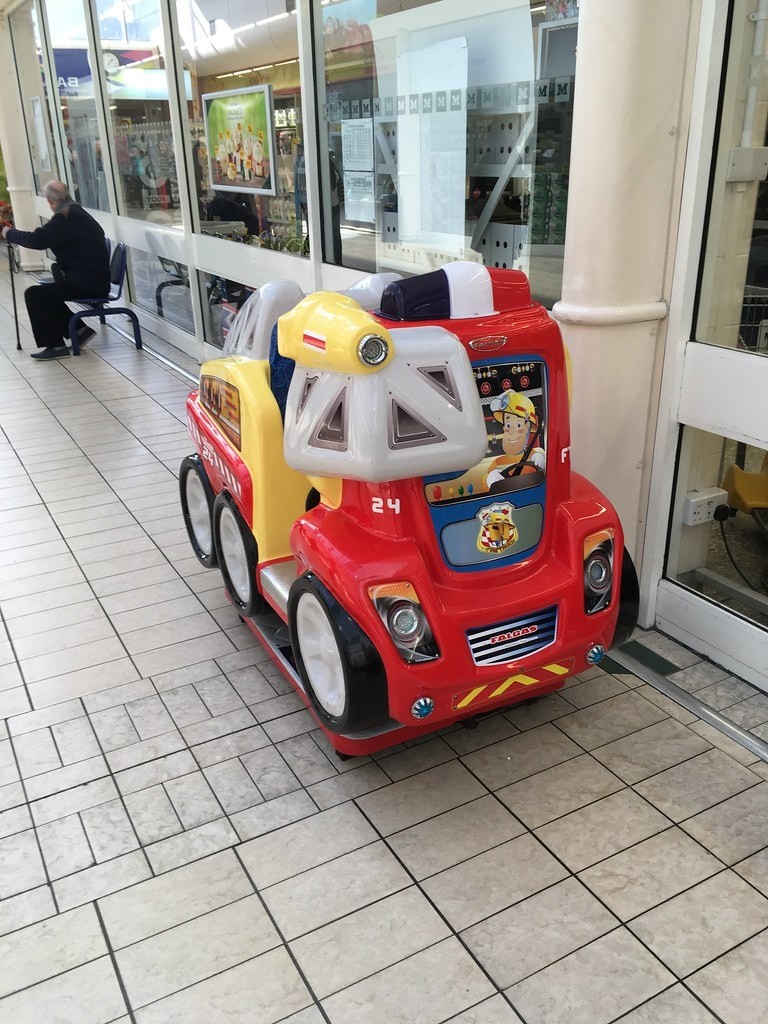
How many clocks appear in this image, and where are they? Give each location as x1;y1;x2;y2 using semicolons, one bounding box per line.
103;51;120;75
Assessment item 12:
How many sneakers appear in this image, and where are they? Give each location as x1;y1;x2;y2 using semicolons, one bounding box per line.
71;325;97;351
30;346;71;360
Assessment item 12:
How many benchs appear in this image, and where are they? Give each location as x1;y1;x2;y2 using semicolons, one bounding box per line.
37;237;142;357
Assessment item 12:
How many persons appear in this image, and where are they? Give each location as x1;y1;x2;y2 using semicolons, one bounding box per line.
205;191;257;310
297;145;343;267
2;180;112;361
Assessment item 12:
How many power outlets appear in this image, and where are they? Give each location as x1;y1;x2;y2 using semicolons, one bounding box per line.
682;486;728;527
